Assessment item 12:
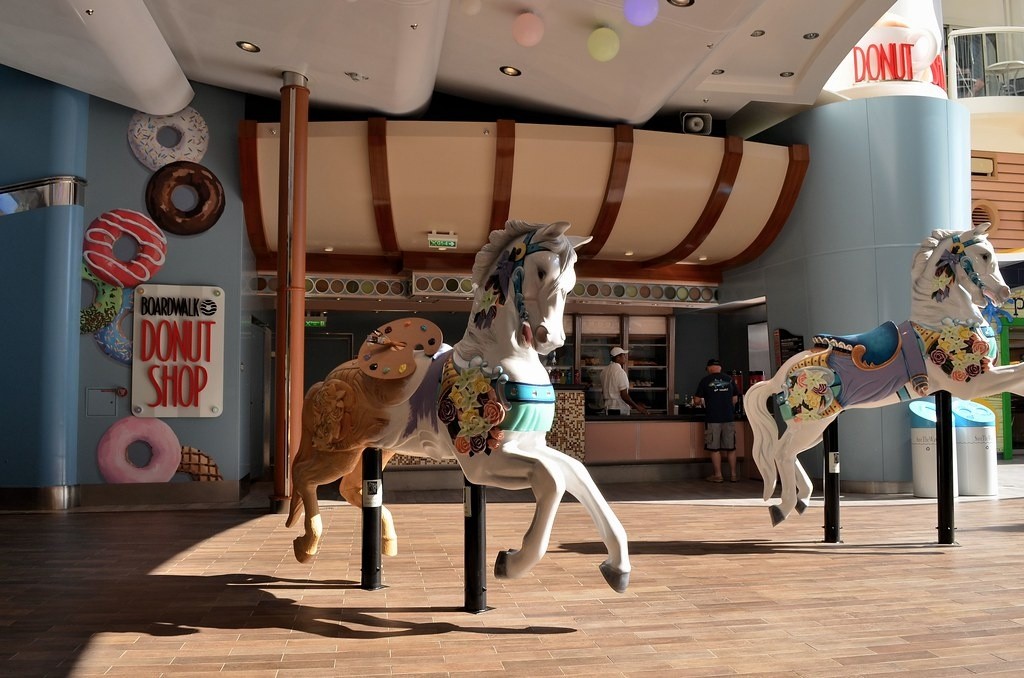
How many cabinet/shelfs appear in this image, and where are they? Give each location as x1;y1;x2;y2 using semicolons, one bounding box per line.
538;334;668;415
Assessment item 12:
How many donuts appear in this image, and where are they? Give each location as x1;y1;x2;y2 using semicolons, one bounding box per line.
77;104;221;483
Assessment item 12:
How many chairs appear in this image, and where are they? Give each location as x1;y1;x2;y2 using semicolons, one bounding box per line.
958;78;977;98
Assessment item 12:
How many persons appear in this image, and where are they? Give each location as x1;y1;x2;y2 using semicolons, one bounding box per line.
695;359;738;482
600;348;647;416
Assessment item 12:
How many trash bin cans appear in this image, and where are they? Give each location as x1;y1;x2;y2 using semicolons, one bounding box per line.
908;401;960;498
952;400;998;496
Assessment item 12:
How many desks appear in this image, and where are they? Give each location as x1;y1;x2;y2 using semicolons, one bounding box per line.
987;60;1024;96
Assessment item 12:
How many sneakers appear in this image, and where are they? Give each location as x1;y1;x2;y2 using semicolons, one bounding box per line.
731;476;740;483
707;474;724;483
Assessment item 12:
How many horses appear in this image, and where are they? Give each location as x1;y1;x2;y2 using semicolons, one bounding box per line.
742;223;1023;526
286;219;632;593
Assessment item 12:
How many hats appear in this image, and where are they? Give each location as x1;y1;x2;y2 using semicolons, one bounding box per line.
705;359;722;371
609;347;629;357
